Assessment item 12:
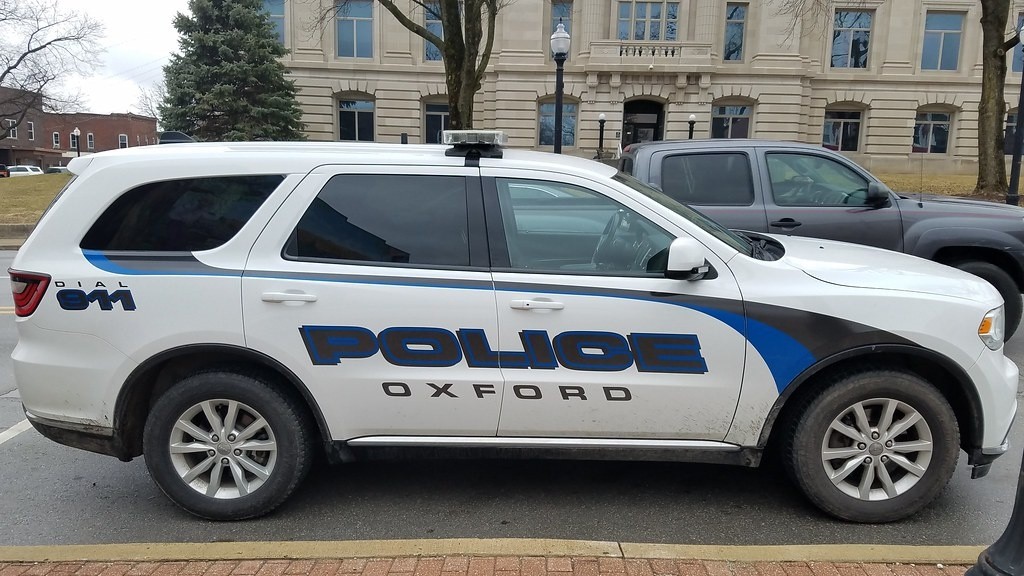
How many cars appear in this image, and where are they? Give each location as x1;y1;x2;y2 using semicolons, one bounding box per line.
0;164;10;178
444;182;569;199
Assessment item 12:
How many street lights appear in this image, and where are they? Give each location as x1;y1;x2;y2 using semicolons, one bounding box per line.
549;16;569;156
687;114;696;137
598;114;605;151
74;126;82;157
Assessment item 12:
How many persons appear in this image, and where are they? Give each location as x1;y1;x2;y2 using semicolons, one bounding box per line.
774;176;814;203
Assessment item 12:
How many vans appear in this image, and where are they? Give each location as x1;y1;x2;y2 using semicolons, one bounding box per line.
6;165;69;177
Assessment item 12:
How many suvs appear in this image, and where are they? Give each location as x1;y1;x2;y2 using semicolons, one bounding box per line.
11;129;1019;524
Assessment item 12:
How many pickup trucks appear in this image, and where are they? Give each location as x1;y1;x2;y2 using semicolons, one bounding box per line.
508;138;1024;350
